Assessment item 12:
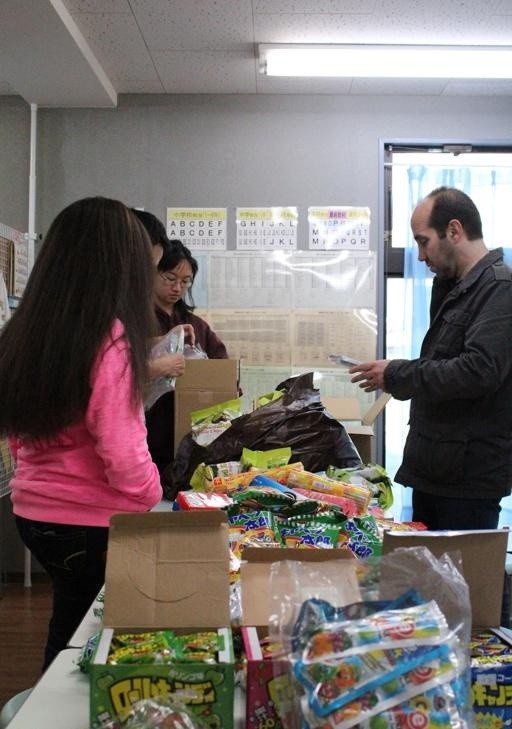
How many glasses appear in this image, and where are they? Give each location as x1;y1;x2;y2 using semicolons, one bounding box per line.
156;270;193;289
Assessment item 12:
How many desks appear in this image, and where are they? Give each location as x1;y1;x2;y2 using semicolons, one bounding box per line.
6;647;245;729
65;487;178;650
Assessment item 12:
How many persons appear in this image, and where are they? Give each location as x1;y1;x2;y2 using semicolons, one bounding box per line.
123;200;198;382
343;187;511;532
0;193;164;672
143;235;235;476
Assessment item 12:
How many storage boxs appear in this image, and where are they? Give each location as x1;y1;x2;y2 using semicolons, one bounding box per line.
173;357;242;458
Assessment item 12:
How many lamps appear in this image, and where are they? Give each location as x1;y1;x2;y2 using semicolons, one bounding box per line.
257;42;512;82
